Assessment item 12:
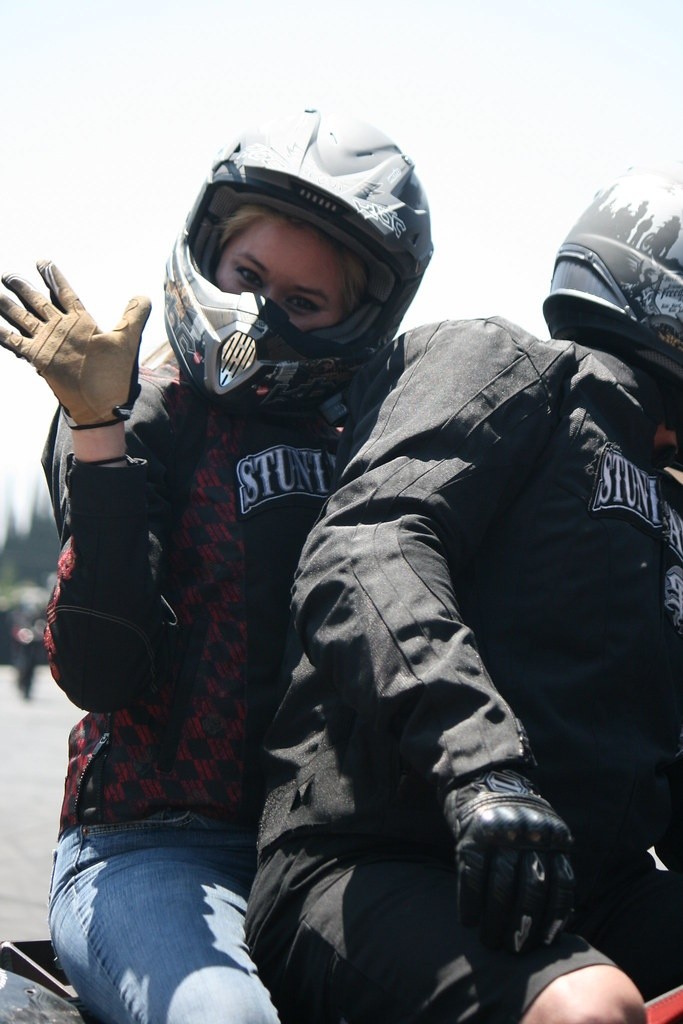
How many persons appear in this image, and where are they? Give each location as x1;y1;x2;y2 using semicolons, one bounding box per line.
2;112;434;1023
12;591;40;699
251;151;681;1023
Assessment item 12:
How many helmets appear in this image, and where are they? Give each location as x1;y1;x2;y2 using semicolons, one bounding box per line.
164;108;435;408
542;156;683;351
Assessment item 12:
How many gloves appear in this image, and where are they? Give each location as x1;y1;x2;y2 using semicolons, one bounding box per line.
444;762;580;957
0;257;151;430
